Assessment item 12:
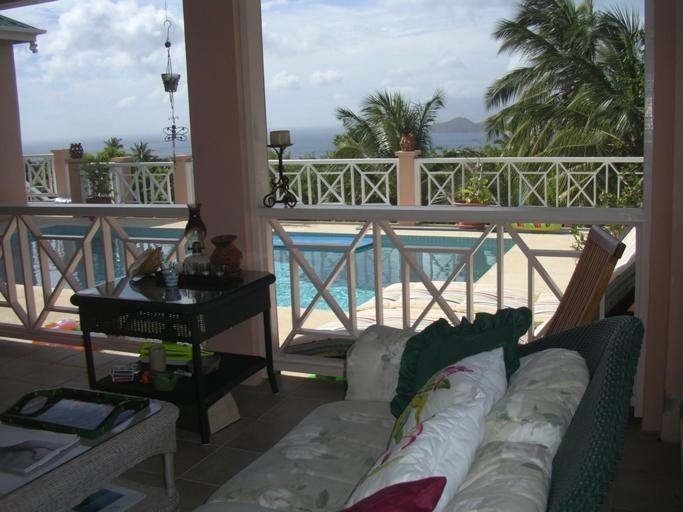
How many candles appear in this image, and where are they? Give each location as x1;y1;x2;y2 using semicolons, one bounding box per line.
270;130;290;145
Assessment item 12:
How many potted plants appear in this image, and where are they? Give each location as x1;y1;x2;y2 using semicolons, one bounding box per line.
453;176;493;231
366;90;421;151
82;163;116;220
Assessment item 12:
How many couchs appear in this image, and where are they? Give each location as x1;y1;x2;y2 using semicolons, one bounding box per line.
193;315;646;512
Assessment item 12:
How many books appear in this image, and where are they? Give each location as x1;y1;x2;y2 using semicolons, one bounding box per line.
1;422;81;474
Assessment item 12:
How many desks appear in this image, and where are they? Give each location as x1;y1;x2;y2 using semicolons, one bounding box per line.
0;386;180;512
70;272;279;441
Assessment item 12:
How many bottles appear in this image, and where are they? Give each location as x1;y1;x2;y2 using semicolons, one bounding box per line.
150;339;166;372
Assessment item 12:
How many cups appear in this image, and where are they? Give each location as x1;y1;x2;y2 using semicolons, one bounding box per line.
163;288;182;302
162;270;179;287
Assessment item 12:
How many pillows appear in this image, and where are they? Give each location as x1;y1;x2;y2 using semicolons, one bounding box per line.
336;347;508;512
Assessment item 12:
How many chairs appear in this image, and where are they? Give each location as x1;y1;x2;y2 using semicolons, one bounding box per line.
544;224;626;337
